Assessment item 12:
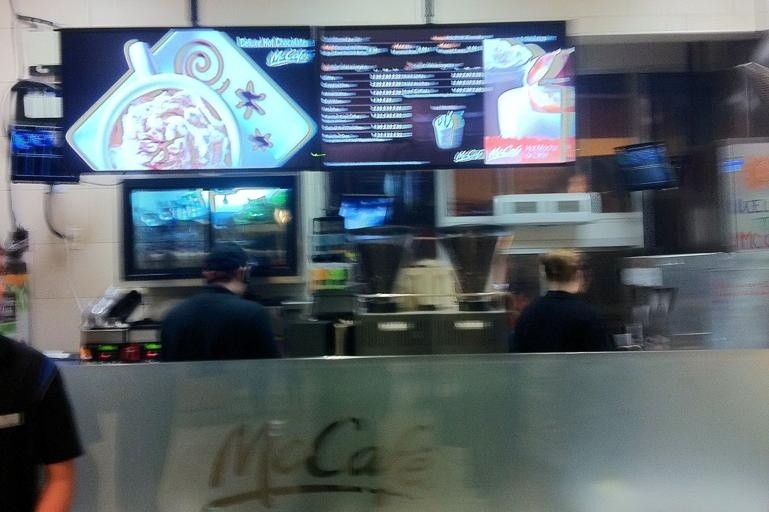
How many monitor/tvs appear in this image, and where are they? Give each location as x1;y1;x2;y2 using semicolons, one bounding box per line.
118;174;298;281
9;124;79;185
337;194;403;231
614;142;678;191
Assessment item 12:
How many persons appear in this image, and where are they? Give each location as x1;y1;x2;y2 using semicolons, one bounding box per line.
159;243;281;362
512;248;613;352
0;248;84;512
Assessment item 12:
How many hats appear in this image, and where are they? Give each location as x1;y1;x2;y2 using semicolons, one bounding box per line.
201;240;259;273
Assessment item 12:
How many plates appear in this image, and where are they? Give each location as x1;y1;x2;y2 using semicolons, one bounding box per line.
105;75;240;171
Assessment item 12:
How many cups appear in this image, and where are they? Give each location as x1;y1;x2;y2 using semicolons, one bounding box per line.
434;117;465;150
